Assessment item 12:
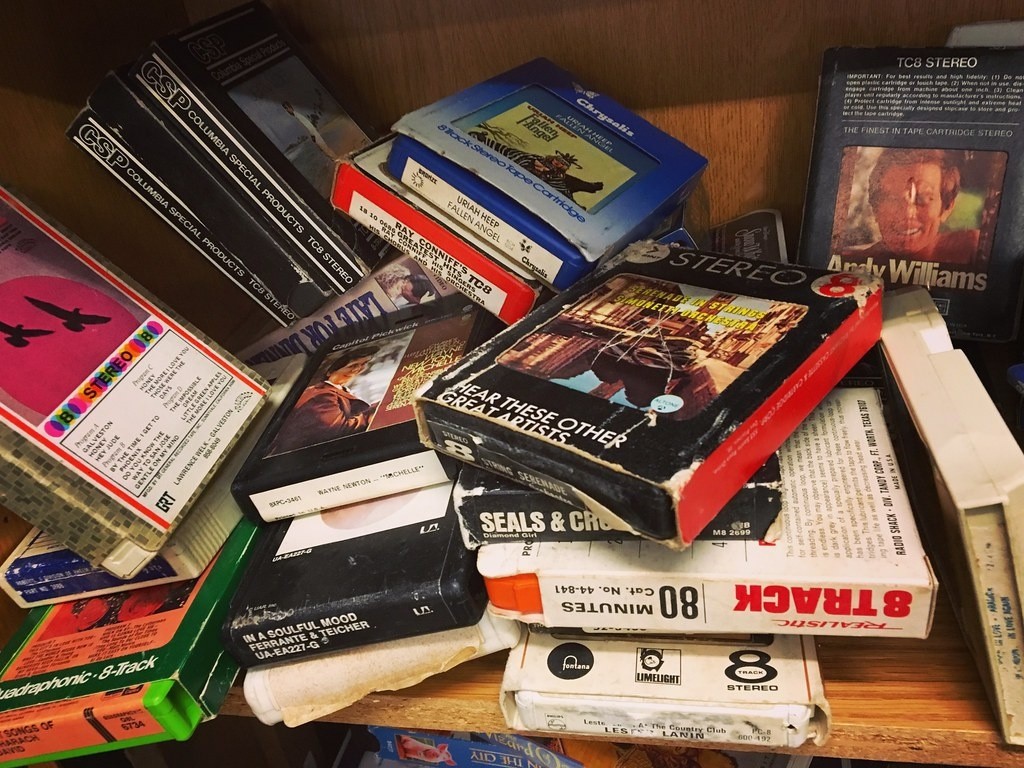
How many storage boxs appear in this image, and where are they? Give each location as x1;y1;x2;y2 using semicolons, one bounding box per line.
0;0;1023;767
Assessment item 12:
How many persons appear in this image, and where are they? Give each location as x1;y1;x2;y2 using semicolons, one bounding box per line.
837;149;981;267
264;346;381;457
280;100;340;164
